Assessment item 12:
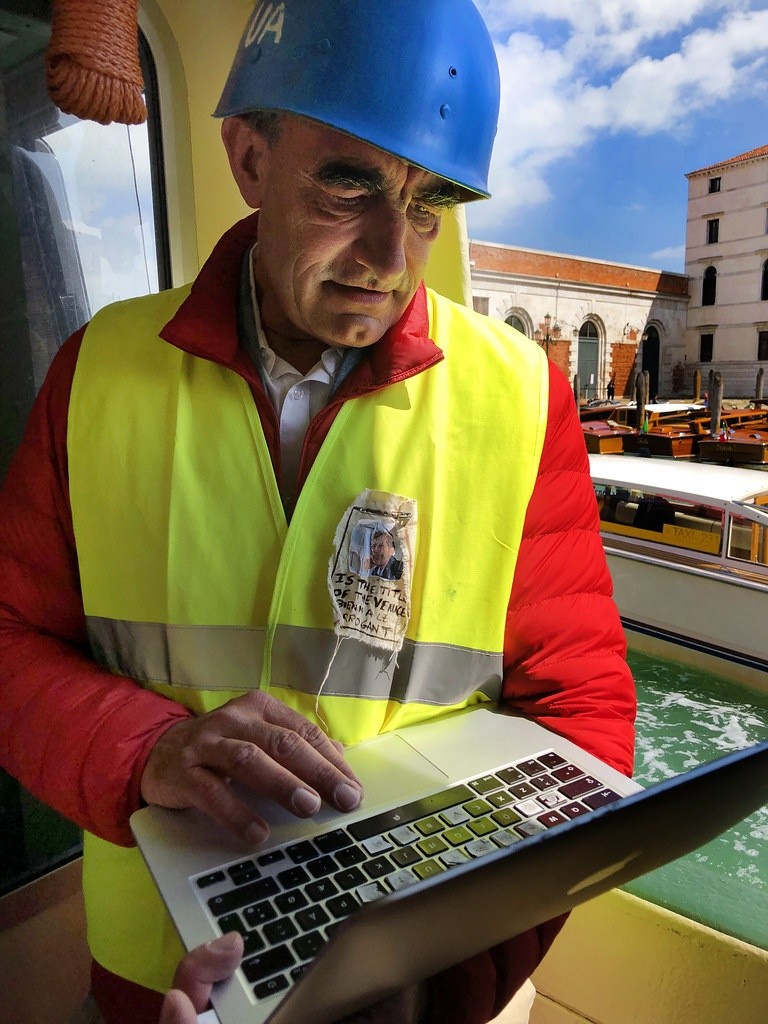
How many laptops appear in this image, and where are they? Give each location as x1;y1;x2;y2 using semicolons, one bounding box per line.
128;699;768;1024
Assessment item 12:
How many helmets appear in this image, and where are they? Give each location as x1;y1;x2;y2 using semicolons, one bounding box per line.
210;1;499;204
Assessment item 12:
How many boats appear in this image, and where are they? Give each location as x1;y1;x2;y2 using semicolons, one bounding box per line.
583;453;768;702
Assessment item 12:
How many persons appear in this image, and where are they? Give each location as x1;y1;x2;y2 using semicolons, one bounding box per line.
365;528;403;580
607;379;616;401
1;0;639;1024
652;394;659;404
703;394;709;407
